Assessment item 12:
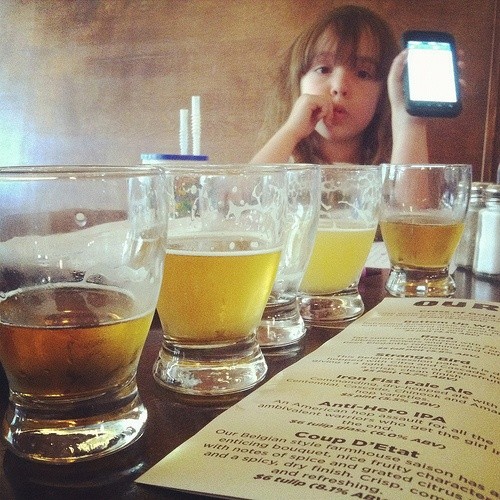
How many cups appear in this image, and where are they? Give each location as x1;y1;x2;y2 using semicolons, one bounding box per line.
138;154;381;395
0;166;175;466
379;163;472;299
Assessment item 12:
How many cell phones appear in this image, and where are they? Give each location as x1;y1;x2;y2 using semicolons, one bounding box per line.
401;30;462;118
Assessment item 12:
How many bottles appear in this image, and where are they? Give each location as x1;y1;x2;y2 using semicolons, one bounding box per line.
454;182;500;279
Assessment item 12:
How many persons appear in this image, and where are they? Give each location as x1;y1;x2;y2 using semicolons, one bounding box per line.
225;6;466;241
